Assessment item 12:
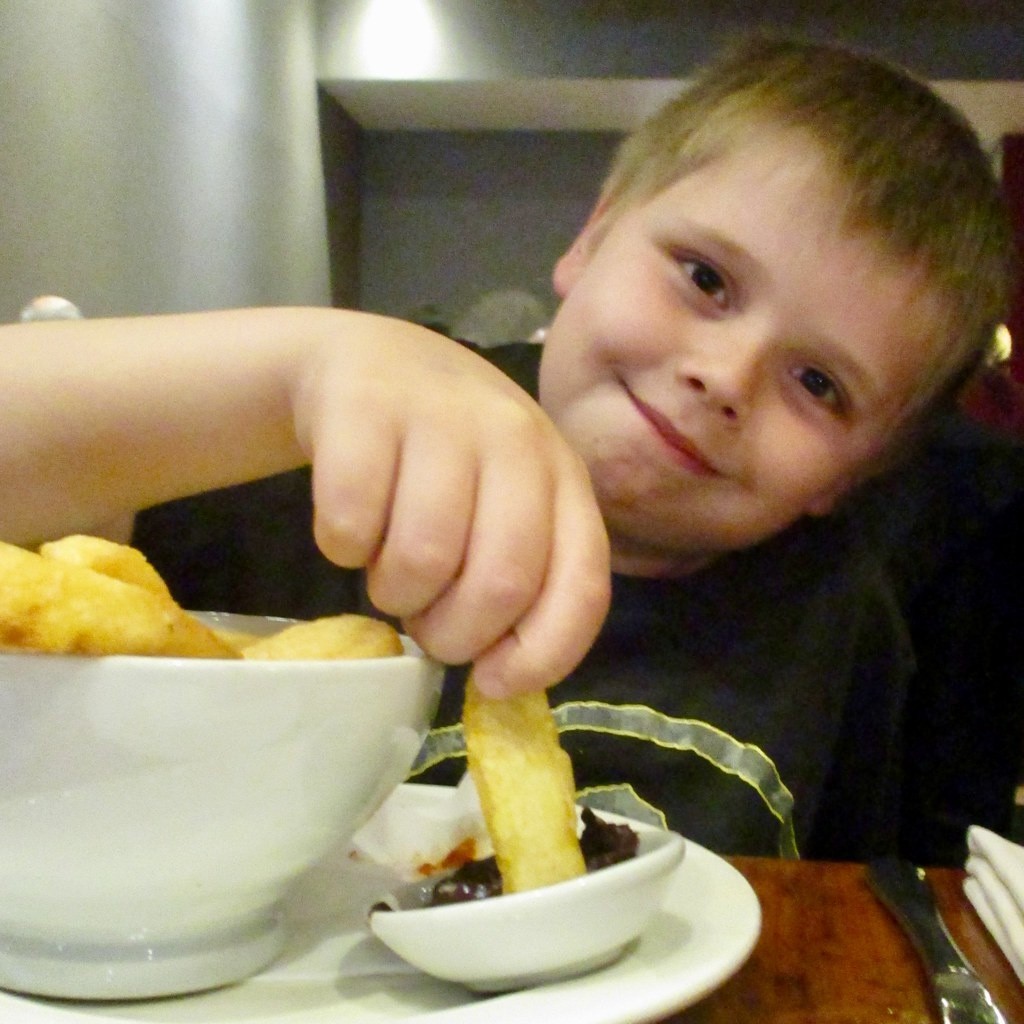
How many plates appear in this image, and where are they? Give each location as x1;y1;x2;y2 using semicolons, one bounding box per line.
0;781;761;1024
368;828;685;995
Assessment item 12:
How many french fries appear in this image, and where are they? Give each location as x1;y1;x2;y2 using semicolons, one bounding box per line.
0;531;403;666
463;656;585;895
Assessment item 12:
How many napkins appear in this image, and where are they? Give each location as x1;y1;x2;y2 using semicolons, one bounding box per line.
963;825;1024;986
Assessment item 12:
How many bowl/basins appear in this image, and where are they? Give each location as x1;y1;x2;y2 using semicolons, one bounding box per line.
1;612;444;1001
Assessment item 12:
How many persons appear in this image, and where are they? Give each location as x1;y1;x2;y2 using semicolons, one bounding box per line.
1;38;1024;856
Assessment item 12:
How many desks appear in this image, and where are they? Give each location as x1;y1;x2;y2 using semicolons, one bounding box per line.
661;854;1024;1024
